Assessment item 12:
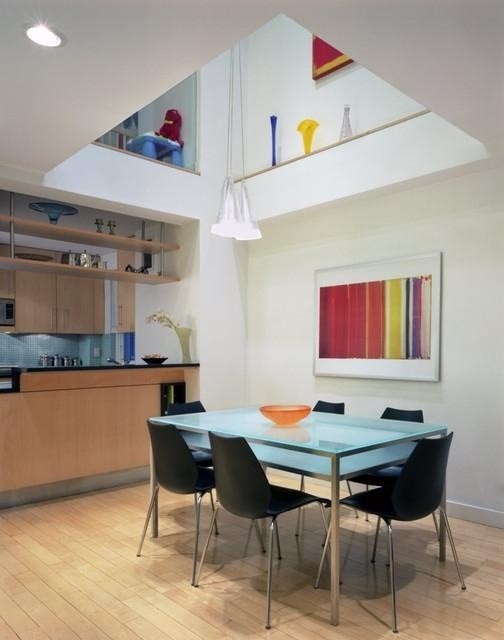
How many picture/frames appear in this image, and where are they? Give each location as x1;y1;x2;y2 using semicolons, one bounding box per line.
312;249;442;380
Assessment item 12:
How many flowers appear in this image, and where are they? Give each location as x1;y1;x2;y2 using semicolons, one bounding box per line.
150;311;179;328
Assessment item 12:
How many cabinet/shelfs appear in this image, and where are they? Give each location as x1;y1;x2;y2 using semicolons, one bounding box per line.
0;192;180;283
0;271;105;335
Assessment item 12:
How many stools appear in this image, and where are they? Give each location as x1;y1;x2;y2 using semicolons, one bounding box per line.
126;133;183;168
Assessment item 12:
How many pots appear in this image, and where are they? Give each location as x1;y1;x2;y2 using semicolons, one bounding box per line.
62;355;72;366
53;353;62;366
72;356;81;366
40;351;53;366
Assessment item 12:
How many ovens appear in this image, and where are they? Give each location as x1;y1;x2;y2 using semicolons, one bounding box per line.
0;363;20;393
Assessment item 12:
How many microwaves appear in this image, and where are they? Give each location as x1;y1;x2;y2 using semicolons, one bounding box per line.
0;299;14;326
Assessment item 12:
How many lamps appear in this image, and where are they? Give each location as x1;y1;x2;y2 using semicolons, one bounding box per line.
209;44;261;241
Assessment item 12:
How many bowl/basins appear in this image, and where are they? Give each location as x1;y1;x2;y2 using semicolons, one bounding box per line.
260;405;312;427
142;357;168;364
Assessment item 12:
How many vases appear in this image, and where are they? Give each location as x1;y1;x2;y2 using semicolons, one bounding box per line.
175;328;191;364
